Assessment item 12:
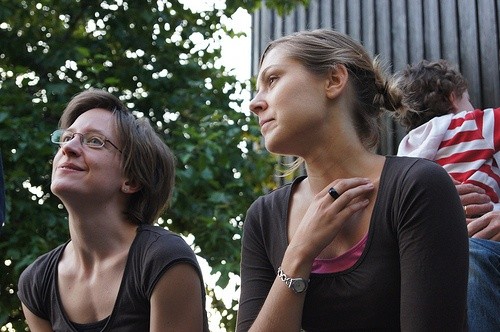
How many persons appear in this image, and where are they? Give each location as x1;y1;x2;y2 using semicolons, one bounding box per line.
17;89;212;332
236;29;500;332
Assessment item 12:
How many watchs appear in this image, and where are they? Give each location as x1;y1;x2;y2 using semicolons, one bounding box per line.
277;268;310;295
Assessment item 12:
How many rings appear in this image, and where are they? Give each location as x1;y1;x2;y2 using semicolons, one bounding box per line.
327;187;340;200
464;206;468;216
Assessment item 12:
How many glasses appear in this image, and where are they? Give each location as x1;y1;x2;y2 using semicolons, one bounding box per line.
50;129;127;162
